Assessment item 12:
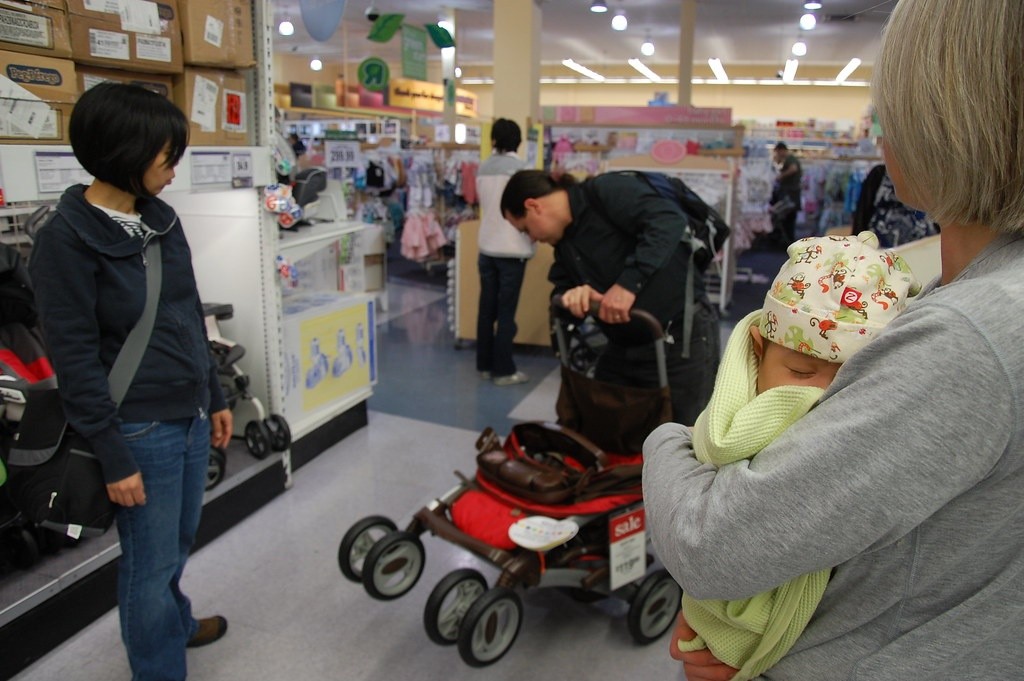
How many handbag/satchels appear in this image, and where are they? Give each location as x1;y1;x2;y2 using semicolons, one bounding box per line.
3;396;117;530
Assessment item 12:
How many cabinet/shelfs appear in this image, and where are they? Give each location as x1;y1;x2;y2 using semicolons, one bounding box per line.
290;102;893;351
0;0;294;681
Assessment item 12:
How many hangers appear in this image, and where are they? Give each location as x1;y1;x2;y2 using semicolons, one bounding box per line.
403;208;440;217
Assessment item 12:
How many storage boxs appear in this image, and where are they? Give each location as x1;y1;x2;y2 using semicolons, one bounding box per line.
0;0;256;146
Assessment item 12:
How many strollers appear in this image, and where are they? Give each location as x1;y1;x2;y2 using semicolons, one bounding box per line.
337;290;683;668
192;300;293;493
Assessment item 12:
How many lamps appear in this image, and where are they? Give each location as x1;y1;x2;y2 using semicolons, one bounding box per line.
804;0;823;9
588;0;609;13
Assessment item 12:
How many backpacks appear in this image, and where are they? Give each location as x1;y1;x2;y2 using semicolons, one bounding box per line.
583;165;732;277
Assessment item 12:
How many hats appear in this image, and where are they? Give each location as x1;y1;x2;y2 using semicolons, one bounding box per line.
759;231;923;362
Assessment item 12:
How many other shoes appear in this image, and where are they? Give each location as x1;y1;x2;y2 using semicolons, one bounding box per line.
184;616;227;648
492;370;530;386
481;370;491;379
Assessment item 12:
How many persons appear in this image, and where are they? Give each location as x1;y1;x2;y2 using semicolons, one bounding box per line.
774;144;800;242
676;231;922;680
28;84;233;680
499;170;719;426
643;0;1024;681
475;118;538;385
290;134;305;154
853;165;941;246
